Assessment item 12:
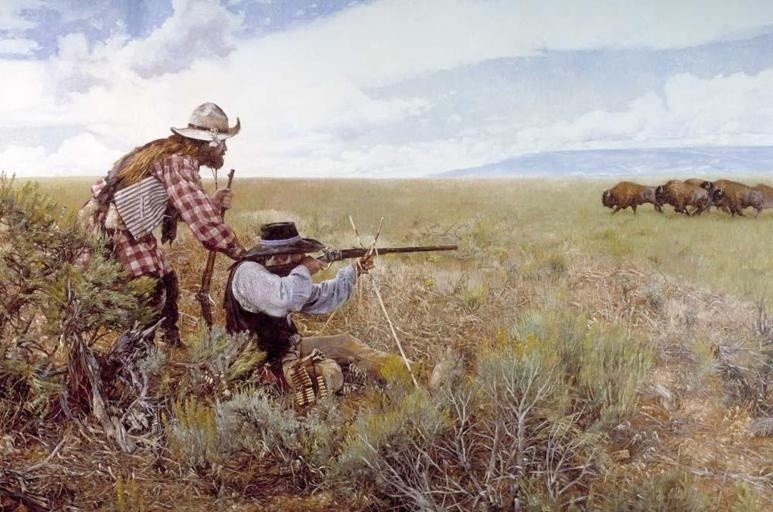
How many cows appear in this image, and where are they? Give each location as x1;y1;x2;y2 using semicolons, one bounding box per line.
600;177;773;219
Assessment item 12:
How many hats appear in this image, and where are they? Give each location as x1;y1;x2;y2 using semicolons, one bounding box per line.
170;102;242;142
237;220;326;258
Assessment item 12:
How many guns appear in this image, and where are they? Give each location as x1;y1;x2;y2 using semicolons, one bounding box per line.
264;244;457;277
196;169;235;329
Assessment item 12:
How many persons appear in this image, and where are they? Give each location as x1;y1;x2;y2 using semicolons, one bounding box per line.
68;99;248;364
217;220;467;397
